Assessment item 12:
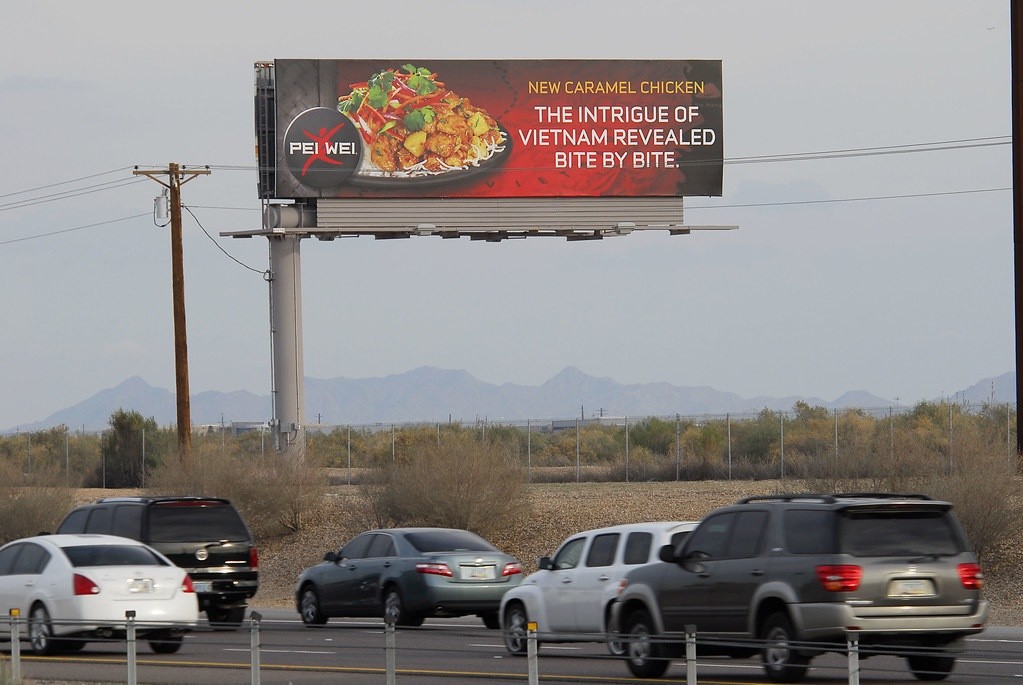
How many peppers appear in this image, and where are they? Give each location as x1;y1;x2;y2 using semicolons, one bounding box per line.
349;72;450;144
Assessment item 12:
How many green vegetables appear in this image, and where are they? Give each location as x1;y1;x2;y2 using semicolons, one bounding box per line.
337;63;435;137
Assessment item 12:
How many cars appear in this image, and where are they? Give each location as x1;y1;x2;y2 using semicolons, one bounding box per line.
0;533;199;653
295;527;519;628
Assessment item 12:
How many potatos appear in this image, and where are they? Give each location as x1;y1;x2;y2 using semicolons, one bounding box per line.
404;111;489;166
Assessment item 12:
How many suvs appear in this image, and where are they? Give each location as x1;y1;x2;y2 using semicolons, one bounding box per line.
38;496;261;629
617;489;988;681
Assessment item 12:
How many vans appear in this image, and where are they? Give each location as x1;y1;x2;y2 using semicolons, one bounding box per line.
498;521;702;653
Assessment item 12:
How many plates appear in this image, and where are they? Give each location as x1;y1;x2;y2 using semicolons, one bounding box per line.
347;114;513;191
